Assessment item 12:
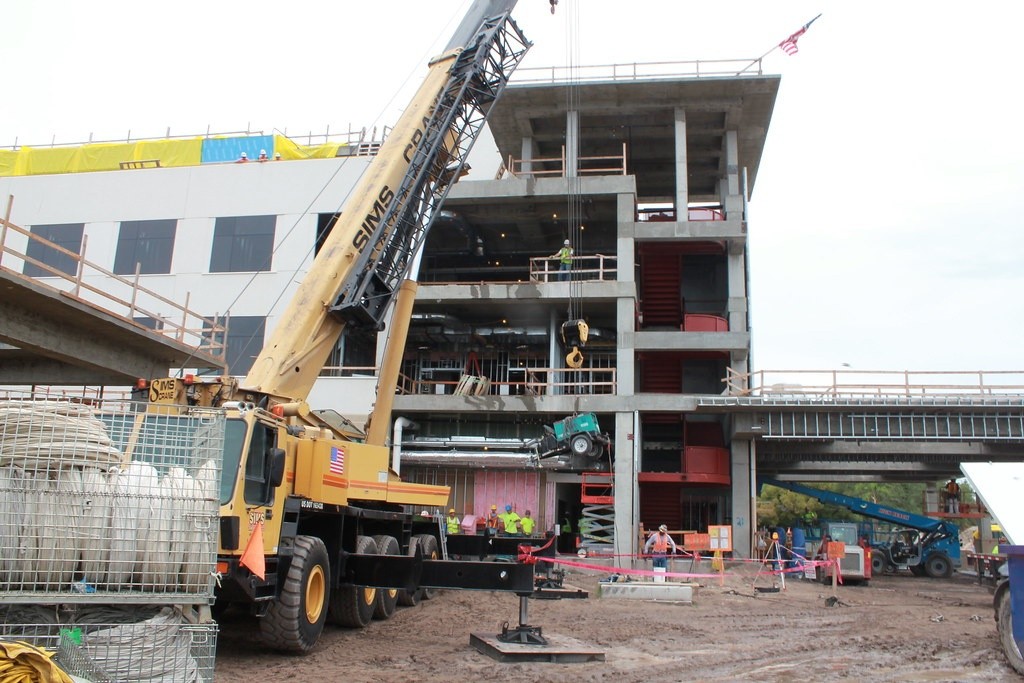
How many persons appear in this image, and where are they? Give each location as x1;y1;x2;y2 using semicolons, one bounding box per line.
643;524;676;582
484;505;500;537
801;506;816;537
577;508;591;551
446;508;460;533
554;239;574;281
258;149;268;162
991;535;1007;554
520;509;535;537
234;152;250;163
273;152;283;160
559;511;572;553
497;504;522;537
945;479;959;513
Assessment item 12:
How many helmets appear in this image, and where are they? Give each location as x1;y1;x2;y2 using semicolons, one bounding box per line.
491;505;497;510
506;506;511;510
449;509;455;513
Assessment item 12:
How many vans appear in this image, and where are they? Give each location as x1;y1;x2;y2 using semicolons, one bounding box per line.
959;524;1009;556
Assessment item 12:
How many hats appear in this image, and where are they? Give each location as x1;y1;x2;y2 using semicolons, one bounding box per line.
563;239;570;245
658;525;667;533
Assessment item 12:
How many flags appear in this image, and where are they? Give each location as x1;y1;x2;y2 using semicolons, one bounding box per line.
778;20;813;56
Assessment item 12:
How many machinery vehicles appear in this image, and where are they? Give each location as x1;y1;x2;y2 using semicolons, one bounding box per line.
812;520;874;586
85;0;593;653
961;458;1024;675
755;472;963;579
